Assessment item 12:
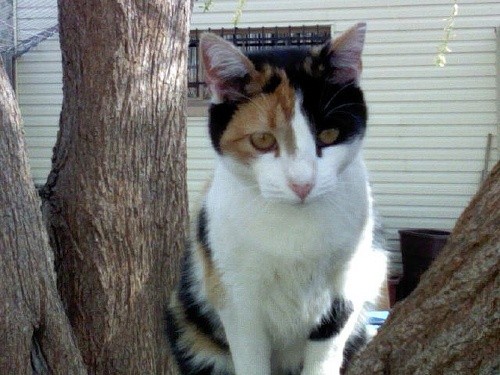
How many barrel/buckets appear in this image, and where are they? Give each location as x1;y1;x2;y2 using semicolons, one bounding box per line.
399;227;452;297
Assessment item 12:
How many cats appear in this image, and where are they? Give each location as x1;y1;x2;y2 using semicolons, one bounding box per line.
159;21;393;375
